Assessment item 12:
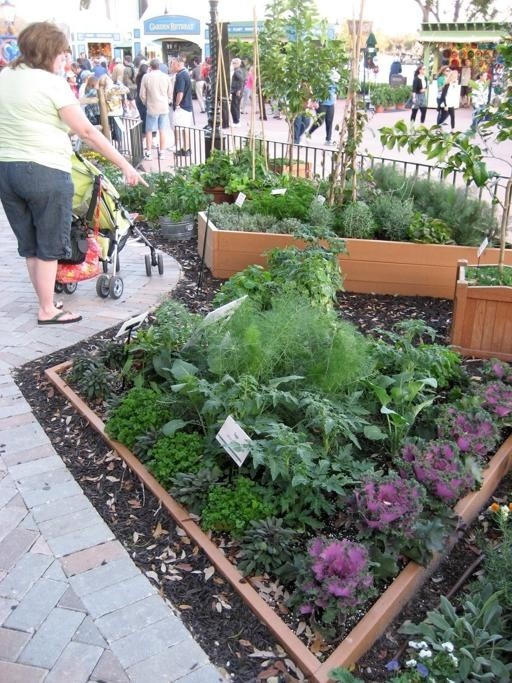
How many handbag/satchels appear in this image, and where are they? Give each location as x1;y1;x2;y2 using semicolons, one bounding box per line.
55;237;100;283
58;217;88;264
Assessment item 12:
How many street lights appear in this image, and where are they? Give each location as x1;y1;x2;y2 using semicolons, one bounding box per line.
363;31;381;107
0;0;17;34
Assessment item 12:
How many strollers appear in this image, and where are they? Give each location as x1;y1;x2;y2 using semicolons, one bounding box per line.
51;140;166;301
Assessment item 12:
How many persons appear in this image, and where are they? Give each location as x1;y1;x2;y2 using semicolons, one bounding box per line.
436;59;474;133
4;41;15;62
59;53;211;158
293;70;340;146
411;66;428;123
389;59;402;85
1;22;149;323
471;72;490;133
230;58;254;124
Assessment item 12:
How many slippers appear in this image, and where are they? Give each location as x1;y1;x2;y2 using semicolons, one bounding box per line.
53;301;63;309
37;310;82;324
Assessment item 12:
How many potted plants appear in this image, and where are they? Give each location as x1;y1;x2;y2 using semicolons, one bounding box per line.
143;142;268;242
370;82;414;113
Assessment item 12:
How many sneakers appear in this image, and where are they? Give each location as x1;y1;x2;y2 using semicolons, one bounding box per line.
143;151;151;160
